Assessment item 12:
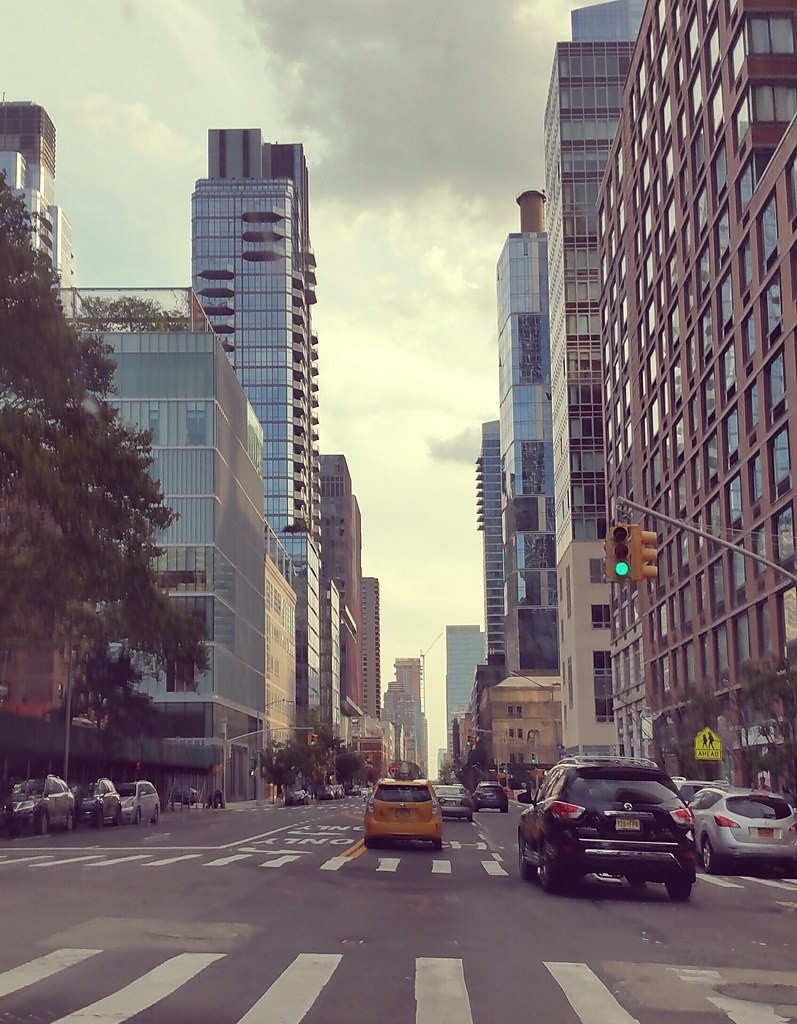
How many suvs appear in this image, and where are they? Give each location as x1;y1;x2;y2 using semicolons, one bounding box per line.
518;754;697;900
686;785;797;873
0;773;74;836
472;781;509;814
113;780;160;826
66;777;122;830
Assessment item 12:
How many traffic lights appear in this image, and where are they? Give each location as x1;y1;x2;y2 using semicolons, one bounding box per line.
500;770;507;778
308;732;317;749
531;752;535;763
603;522;633;583
250;770;255;776
631;524;658;581
467;736;475;746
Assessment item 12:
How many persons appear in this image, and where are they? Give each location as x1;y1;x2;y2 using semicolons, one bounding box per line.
782;784;797;808
751;777;770;790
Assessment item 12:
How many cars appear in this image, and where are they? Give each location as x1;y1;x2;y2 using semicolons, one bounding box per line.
363;778;446;850
670;776;730;804
168;786;199;806
433;784;474;822
346;785;361;796
317;785;346;800
285;790;309;805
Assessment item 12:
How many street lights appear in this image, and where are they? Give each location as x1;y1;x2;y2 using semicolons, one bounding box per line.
509;672;560;760
256;700;294;806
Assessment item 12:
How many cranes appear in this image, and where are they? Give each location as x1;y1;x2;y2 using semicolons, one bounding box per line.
418;631;444;777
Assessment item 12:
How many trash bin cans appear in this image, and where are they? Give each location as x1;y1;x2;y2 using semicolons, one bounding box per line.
212;789;225;808
